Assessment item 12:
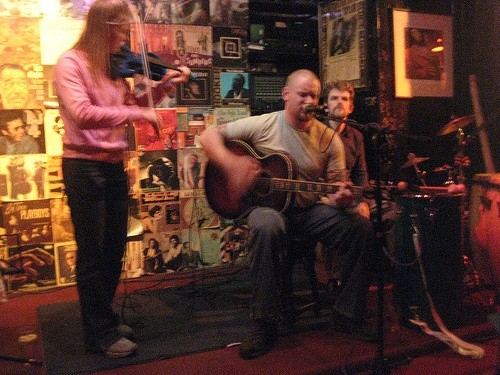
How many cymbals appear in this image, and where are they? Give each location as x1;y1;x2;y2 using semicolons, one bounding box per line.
402;157;430;169
438;114;478;136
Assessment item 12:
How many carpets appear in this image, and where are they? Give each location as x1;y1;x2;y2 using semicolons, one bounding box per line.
37;273;337;375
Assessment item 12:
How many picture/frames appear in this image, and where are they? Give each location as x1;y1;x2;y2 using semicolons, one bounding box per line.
388;7;456;100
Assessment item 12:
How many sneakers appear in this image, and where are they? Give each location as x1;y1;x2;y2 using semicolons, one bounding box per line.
336;313;380;342
239;322;280;359
102;336;138;357
117;323;135;337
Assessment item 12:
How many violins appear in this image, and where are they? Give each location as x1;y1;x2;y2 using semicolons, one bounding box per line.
108;46;194;82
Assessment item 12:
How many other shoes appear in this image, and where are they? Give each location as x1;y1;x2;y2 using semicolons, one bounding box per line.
399;305;421;329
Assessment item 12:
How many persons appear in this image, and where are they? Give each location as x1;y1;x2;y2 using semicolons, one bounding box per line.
200;68;379;358
55;0;191;357
319;79;400;285
0;1;251;288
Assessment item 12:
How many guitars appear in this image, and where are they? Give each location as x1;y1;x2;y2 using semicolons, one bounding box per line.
203;139;399;222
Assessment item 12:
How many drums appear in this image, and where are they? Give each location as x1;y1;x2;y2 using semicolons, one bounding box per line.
468;173;500;289
399;186;467;324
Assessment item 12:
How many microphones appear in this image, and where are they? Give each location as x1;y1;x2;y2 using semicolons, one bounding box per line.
302;104;329;116
